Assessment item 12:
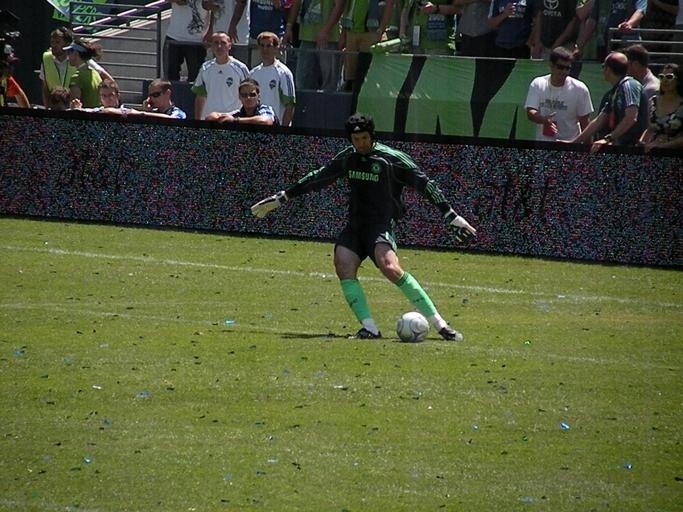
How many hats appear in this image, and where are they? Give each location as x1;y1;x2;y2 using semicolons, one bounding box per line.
62;39;86;53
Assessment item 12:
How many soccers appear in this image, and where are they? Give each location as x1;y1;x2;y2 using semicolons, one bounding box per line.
397;312;429;342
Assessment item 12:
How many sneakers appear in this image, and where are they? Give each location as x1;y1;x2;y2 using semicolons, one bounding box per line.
346;327;382;339
438;324;463;342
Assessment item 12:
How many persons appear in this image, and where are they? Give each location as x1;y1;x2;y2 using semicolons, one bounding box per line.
1;27;185;120
250;113;477;342
524;46;683;155
191;31;296;126
162;0;682;89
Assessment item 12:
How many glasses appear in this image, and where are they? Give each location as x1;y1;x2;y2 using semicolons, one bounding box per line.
239;92;256;97
658;73;676;81
601;64;606;69
148;92;161;98
555;64;571;70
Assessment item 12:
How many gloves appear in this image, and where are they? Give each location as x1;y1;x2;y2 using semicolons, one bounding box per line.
250;190;288;219
444;209;477;244
